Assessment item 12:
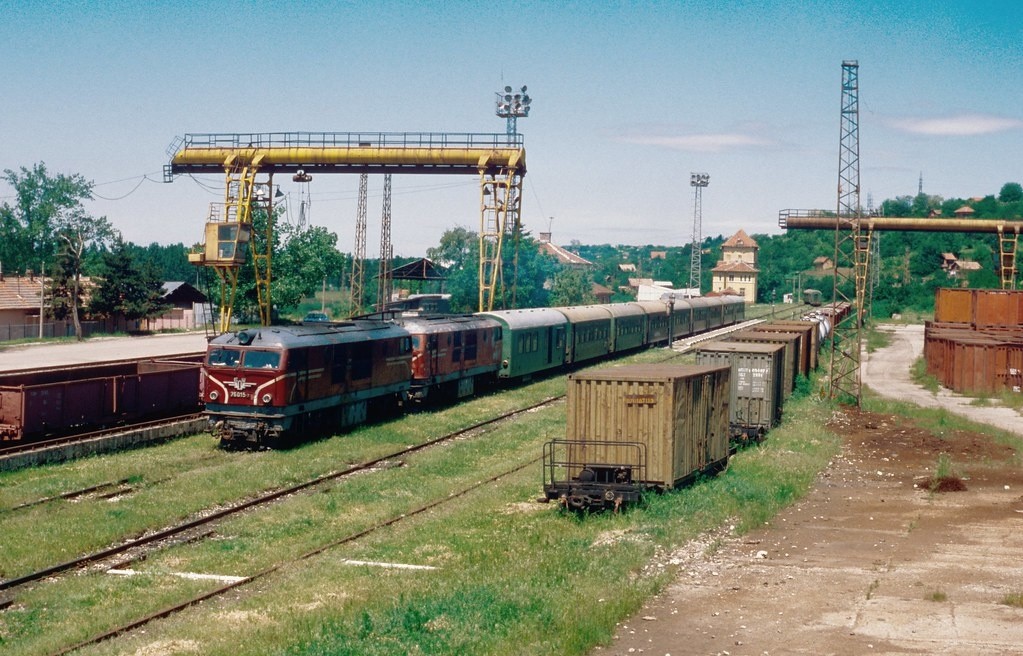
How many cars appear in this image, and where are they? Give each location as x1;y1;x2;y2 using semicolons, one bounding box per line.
303;311;331;323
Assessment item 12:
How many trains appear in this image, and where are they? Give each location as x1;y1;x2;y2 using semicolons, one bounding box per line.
197;288;747;448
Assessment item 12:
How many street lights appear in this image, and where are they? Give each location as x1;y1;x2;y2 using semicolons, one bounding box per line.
494;85;533;256
689;171;710;297
